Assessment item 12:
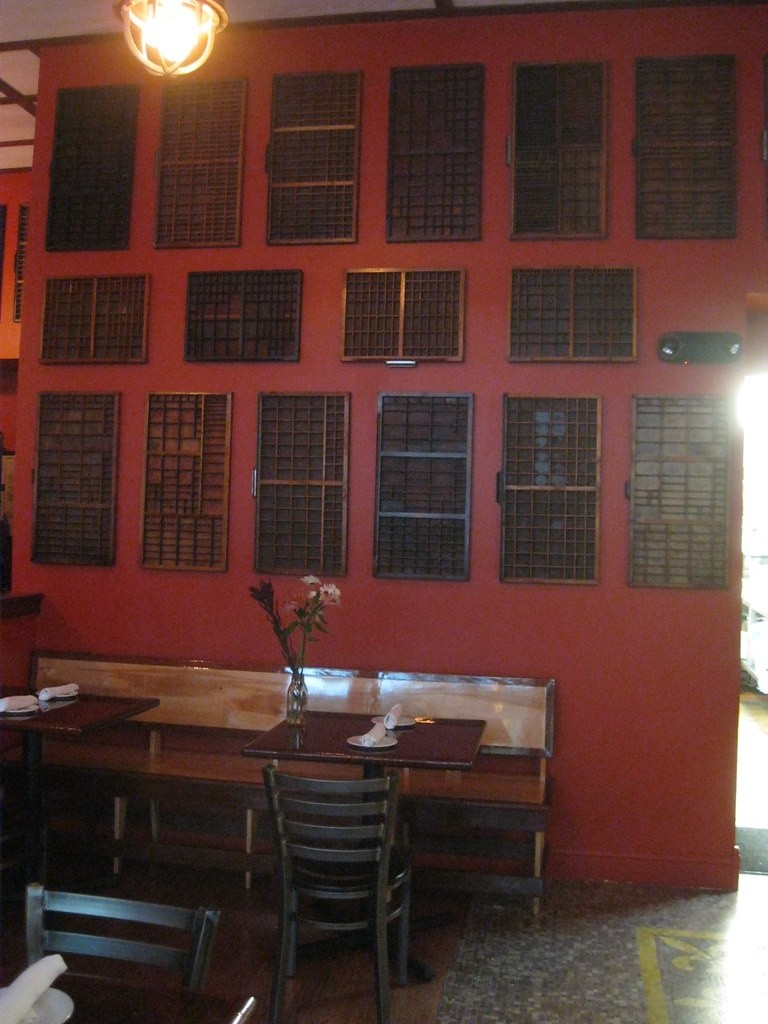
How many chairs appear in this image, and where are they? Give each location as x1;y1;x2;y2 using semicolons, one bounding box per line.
263;765;414;1024
21;881;221;991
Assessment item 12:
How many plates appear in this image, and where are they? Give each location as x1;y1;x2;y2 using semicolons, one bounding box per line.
347;735;398;748
0;986;74;1024
4;704;38;713
370;715;416;726
35;690;78;697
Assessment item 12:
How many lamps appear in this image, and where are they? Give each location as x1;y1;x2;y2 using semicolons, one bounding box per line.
117;0;228;78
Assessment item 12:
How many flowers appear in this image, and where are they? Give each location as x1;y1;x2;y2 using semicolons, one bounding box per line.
249;573;342;724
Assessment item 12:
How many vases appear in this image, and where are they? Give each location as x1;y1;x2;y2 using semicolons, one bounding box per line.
286;672;308;726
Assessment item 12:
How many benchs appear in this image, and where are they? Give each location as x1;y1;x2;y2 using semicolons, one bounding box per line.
26;650;558;917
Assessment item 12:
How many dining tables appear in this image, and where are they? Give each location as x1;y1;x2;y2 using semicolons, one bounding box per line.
0;964;259;1024
0;686;162;932
245;711;486;987
